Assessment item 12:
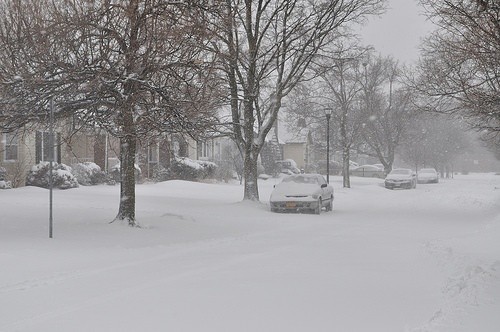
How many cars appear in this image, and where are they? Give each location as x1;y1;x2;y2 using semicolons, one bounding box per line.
341;165;388;179
269;174;335;215
417;168;440;183
374;163;387;174
385;168;416;189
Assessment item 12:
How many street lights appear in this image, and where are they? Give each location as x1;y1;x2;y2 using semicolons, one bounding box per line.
324;104;334;184
